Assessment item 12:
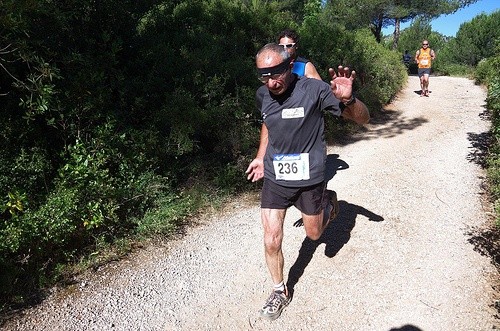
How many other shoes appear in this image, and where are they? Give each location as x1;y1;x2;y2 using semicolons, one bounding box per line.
425;90;429;95
420;92;424;97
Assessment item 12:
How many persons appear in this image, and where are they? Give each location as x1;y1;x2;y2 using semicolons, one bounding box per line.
404;50;411;67
245;29;371;320
415;40;435;96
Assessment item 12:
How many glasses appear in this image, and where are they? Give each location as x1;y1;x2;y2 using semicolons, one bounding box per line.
422;44;428;46
278;43;296;48
258;67;287;84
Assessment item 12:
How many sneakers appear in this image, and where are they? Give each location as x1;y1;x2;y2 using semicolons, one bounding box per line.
261;285;291;320
326;190;339;221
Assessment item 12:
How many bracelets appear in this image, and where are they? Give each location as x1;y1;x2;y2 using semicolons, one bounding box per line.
343;95;355;106
